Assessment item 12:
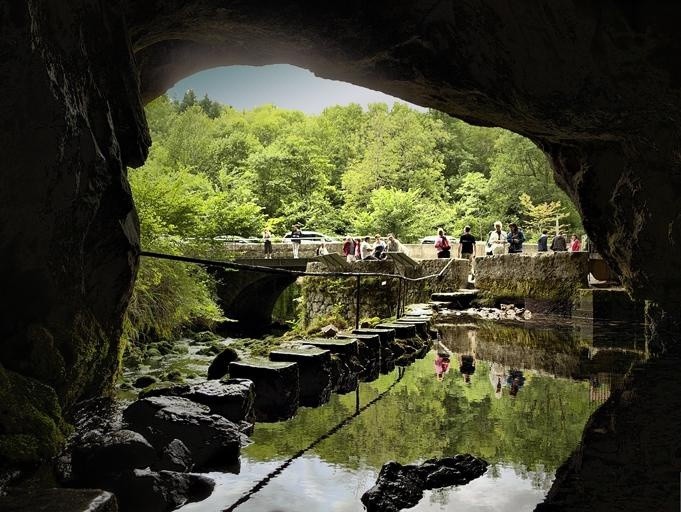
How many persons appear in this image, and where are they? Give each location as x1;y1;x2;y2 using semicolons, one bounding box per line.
317;233;406;263
263;229;274;259
436;349;452;380
551;230;568;253
484;230;495;256
571;233;581;251
506;222;527;253
538;230;548;252
489;362;509;399
489;220;509;254
506;367;527;398
433;228;452;258
458;354;477;384
457;226;477;275
291;225;304;260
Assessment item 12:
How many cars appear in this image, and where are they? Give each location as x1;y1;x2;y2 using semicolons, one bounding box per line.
421;235;455;243
216;235;250;243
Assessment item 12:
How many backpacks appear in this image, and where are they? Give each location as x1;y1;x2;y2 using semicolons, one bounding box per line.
439;237;451;251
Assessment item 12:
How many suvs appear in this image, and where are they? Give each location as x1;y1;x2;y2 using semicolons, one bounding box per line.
282;230;338;244
